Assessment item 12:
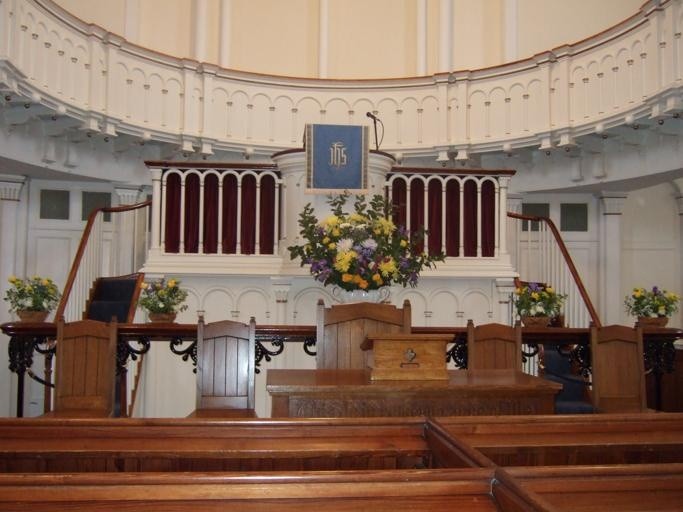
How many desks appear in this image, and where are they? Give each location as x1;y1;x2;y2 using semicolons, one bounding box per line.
265;368;565;419
1;321;683;420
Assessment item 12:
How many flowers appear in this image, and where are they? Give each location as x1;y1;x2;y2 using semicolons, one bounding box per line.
287;190;447;291
623;286;683;317
512;282;567;318
132;277;190;316
4;276;63;314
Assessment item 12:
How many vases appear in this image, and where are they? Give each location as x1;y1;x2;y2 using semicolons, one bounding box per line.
148;311;177;324
331;281;390;305
17;309;48;322
520;316;551;330
637;315;669;327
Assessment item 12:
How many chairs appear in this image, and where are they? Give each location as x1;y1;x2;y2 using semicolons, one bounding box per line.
37;317;118;420
186;318;261;420
466;317;524;372
588;323;656;415
315;297;412;371
